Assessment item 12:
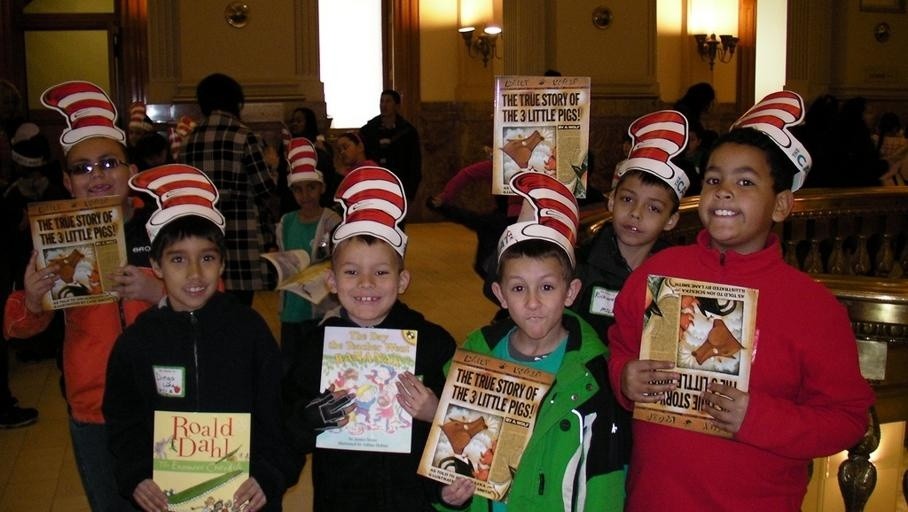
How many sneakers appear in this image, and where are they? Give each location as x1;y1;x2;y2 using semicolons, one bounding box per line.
0;407;38;428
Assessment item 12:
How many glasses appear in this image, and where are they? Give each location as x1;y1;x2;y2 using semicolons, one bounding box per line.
67;157;128;173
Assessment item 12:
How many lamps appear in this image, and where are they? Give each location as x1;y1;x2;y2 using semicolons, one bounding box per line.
455;1;502;68
685;1;741;70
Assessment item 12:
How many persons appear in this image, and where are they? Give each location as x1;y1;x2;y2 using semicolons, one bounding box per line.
0;70;908;512
